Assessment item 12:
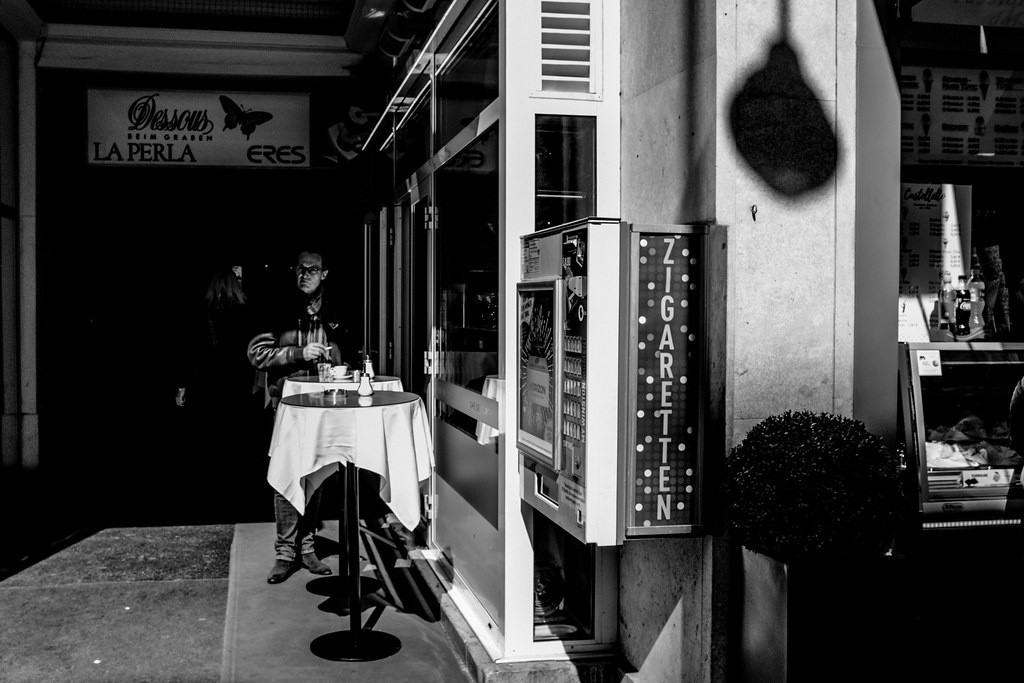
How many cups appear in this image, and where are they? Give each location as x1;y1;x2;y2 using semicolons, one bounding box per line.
332;366;347;375
353;370;360;382
318;362;331;382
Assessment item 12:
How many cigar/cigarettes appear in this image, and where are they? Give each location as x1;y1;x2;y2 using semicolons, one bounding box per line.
323;345;333;353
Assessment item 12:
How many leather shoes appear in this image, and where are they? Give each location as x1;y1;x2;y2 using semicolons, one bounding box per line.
267;560;293;583
295;553;332;575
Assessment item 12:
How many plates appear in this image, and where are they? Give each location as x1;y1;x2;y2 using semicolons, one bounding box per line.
333;375;352;380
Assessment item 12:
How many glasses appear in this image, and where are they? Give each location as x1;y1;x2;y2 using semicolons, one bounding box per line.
296;266;323;275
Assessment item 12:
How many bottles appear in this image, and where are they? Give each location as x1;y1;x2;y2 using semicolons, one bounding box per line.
357;395;373;407
362;360;375;380
938;272;985;336
358;373;373;396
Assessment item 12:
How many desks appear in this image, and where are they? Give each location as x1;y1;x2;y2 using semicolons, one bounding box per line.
267;389;437;663
268;369;405;597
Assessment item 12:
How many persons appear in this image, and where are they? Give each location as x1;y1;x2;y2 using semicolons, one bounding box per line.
174;266;263;517
246;250;360;585
